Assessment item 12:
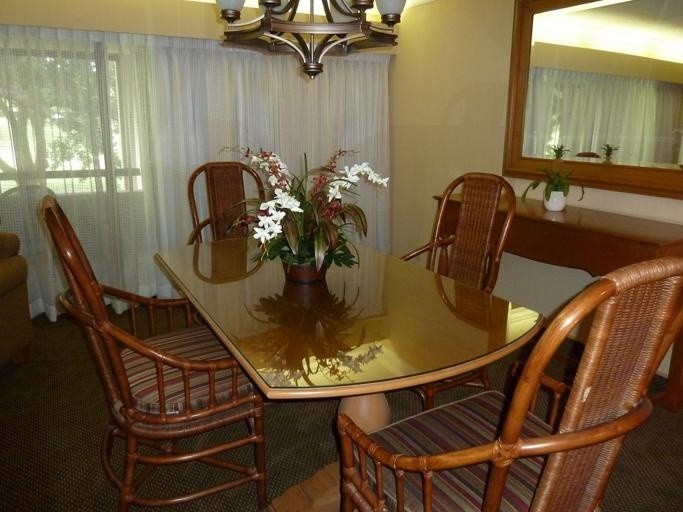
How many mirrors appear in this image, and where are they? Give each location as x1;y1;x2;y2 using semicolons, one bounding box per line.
500;0;683;201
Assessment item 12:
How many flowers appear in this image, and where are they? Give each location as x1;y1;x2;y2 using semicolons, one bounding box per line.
238;282;379;390
216;144;390;272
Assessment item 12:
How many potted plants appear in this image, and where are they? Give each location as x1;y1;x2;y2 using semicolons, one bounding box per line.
521;164;585;212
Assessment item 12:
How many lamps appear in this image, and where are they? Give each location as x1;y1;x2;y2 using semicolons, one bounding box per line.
215;0;406;80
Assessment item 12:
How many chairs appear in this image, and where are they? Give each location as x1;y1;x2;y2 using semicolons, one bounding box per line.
0;232;36;366
436;273;509;332
334;258;681;511
187;162;266;246
44;195;268;512
193;237;266;284
396;170;516;294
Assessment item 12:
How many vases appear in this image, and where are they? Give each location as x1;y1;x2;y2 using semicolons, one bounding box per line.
281;257;328;284
282;283;329;303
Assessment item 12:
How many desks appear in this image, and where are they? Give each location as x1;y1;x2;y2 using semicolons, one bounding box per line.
155;233;543;432
431;194;682;416
